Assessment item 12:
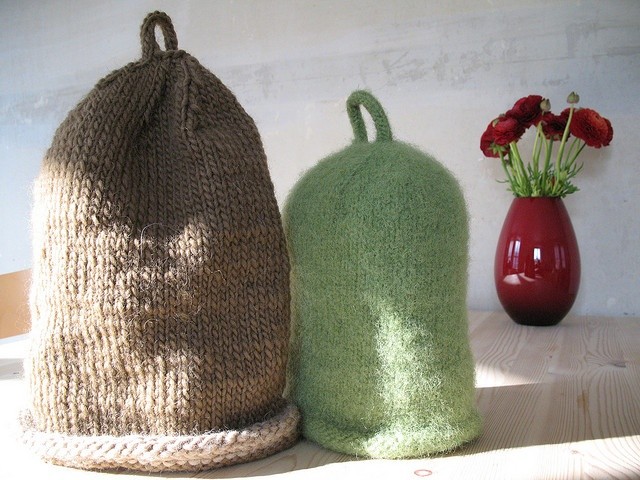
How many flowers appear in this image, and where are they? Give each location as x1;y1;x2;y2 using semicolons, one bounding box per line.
479;90;614;198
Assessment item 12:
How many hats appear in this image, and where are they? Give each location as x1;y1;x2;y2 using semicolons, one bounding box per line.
283;90;483;459
19;11;303;473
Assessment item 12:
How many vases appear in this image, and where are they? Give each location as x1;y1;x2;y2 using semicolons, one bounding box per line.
494;198;581;327
